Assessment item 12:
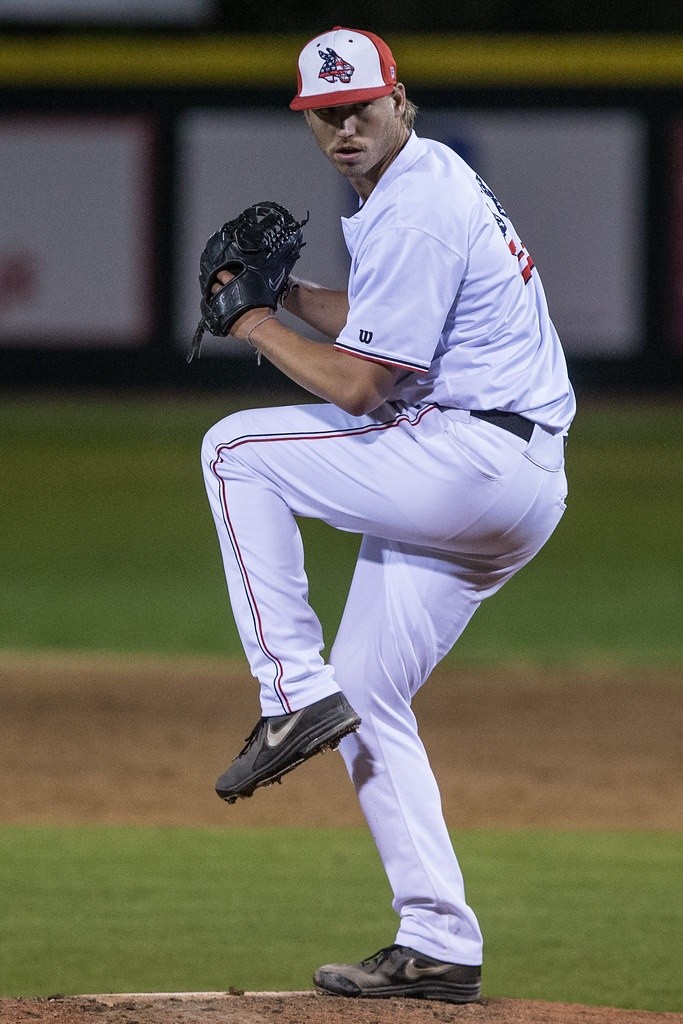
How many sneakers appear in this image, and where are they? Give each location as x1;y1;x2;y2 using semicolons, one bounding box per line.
215;692;361;804
314;945;481;1003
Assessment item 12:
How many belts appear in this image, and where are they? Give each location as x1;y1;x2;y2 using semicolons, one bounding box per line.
437;406;534;443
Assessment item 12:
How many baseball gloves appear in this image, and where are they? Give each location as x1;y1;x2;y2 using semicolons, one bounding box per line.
196;199;308;336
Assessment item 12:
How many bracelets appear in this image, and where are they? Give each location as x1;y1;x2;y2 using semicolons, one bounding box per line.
247;316;276;366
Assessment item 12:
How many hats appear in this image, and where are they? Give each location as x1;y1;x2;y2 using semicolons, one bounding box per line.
289;26;396;110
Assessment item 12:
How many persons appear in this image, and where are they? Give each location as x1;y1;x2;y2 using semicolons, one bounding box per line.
187;25;576;1003
